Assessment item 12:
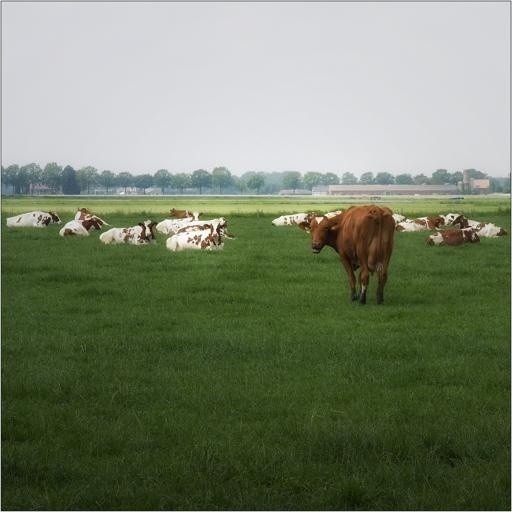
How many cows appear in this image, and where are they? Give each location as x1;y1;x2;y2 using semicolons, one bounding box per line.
393;208;505;237
73;207;109;231
99;220;156;245
157;208;235;252
272;211;342;227
6;209;63;229
298;204;397;306
428;226;481;245
57;219;101;237
